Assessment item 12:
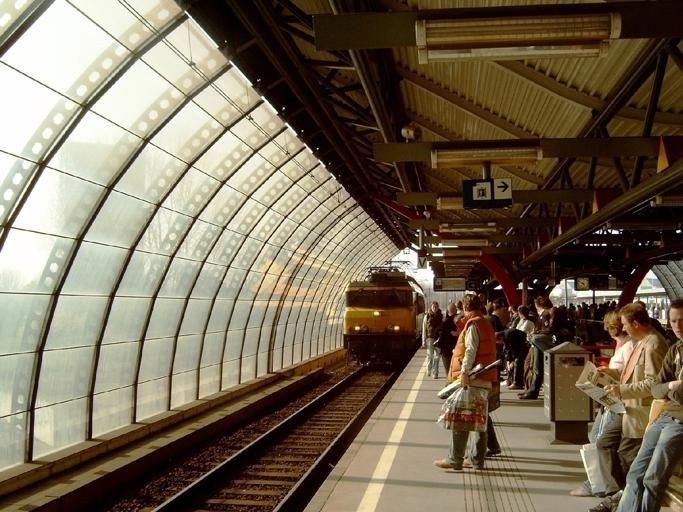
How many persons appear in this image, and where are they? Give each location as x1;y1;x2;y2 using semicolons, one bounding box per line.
494;329;530;390
574;321;589;343
507;303;520;329
422;301;443;379
485;414;503;459
557;300;617;327
484;305;507;331
454;301;463;317
491;298;509;383
615;298;682;512
515;305;535;387
570;312;635;497
442;303;459;374
434;293;498;471
517;295;559;400
588;302;670;512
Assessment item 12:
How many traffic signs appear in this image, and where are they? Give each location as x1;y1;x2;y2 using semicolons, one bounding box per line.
464;180;511;207
434;277;466;293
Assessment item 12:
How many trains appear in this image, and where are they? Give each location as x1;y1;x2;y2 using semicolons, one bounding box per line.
344;270;417;367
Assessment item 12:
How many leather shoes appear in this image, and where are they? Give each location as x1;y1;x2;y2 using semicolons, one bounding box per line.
518;394;537;399
570;489;590;496
506;382;522;389
485;449;501;457
518;392;525;395
433;458;462;470
462;458;482;469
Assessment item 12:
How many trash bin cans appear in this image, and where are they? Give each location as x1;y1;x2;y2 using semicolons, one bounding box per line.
543;343;593;444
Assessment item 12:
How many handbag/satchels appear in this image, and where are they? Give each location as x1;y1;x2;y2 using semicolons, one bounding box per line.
437;385;488;432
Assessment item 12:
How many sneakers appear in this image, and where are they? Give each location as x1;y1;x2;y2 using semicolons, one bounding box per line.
588;502;610;512
426;371;430;376
500;380;509;386
432;374;438;379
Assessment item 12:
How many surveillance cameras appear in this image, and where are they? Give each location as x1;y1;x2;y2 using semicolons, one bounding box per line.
401;125;422;140
